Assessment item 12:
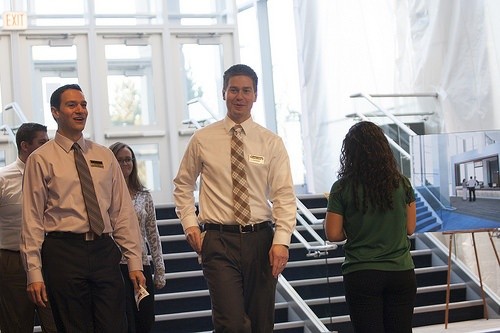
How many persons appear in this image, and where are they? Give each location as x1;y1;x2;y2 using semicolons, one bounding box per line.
462;176;479;202
324;121;416;333
0;123;58;333
172;64;297;333
19;84;150;333
108;142;167;333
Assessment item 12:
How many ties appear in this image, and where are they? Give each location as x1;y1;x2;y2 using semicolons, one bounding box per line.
231;124;251;227
72;142;105;236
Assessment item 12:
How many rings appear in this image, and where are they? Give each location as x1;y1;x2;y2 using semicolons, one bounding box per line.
282;263;285;266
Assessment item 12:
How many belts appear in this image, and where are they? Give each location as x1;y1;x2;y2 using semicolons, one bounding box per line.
45;231;109;241
204;221;272;233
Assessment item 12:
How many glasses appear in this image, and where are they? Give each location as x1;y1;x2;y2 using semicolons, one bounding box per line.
117;158;135;163
